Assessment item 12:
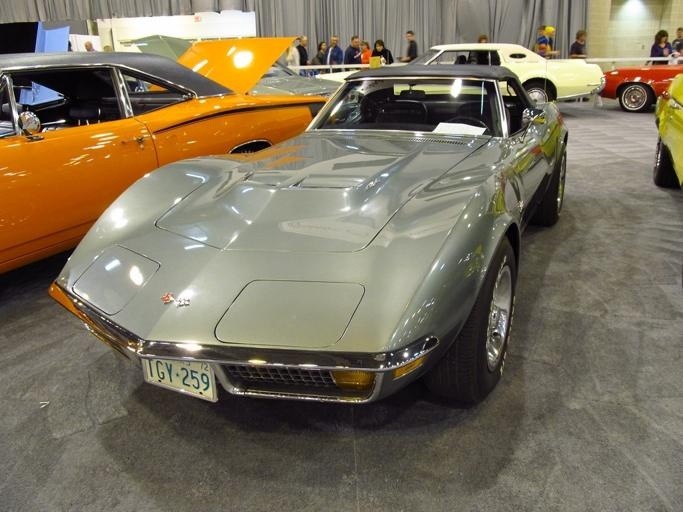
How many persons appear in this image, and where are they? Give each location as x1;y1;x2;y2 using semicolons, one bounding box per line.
85;42;96;52
571;31;588;59
285;35;393;77
479;35;488;43
532;26;553;58
535;26;561;58
400;31;417;62
650;27;683;65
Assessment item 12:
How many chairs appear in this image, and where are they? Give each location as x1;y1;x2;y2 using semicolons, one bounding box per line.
454;102;511;136
68;95;103;125
374;99;428;123
455;55;466;64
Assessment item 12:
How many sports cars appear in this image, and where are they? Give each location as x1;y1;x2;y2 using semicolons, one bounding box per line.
48;63;570;412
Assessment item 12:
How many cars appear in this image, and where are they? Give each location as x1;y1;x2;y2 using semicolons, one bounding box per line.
595;64;683;113
112;34;366;115
0;37;337;275
310;41;605;120
652;71;683;192
